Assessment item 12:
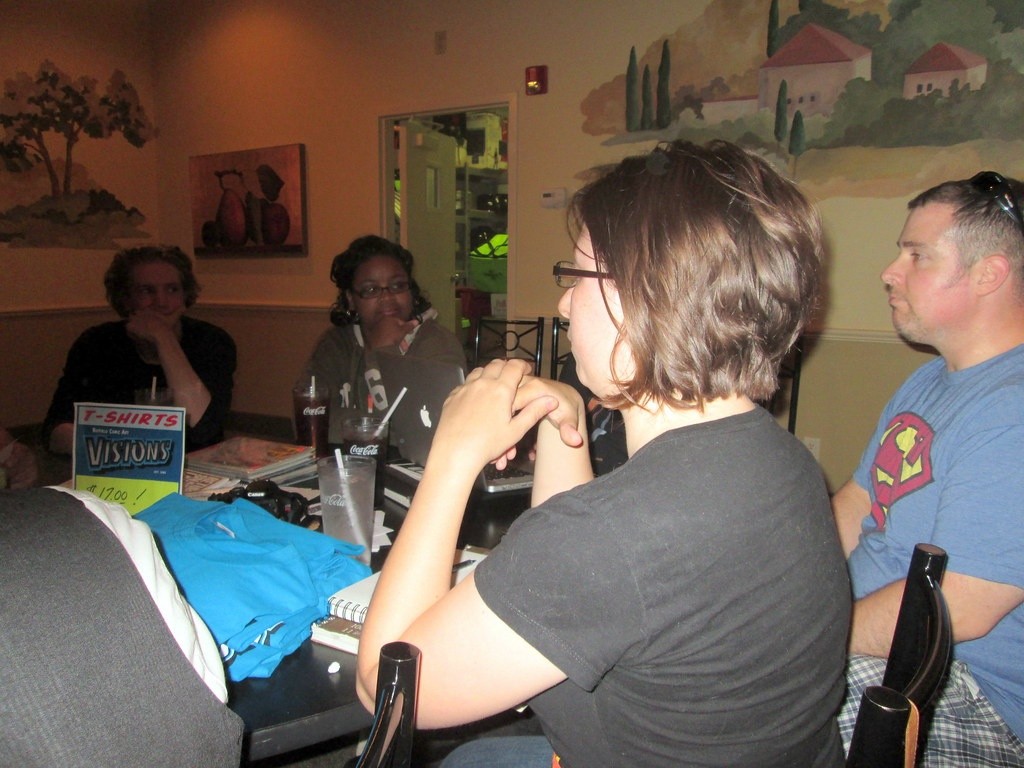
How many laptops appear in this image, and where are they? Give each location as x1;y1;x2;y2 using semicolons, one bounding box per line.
376;350;535;493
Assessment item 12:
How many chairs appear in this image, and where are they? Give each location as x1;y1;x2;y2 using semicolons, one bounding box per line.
346;543;951;767
467;314;574;382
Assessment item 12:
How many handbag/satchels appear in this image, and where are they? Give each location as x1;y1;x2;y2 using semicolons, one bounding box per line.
123;491;375;682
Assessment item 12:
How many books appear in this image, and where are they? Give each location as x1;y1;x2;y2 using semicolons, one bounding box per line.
183;469;243;500
183;434;317;483
310;544;494;655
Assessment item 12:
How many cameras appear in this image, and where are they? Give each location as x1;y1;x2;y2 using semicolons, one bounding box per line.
206;479;308;528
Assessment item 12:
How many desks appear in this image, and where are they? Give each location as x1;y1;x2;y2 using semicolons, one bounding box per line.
29;440;532;766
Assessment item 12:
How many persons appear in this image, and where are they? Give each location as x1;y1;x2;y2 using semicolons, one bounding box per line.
297;235;467;447
353;138;852;766
830;173;1023;766
41;243;237;459
0;486;244;768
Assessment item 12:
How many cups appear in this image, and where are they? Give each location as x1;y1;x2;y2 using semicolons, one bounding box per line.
134;387;174;407
317;455;376;567
292;387;331;457
343;415;390;509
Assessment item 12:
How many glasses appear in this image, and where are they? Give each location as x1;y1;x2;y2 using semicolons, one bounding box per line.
969;168;1024;232
349;281;412;299
552;260;613;288
